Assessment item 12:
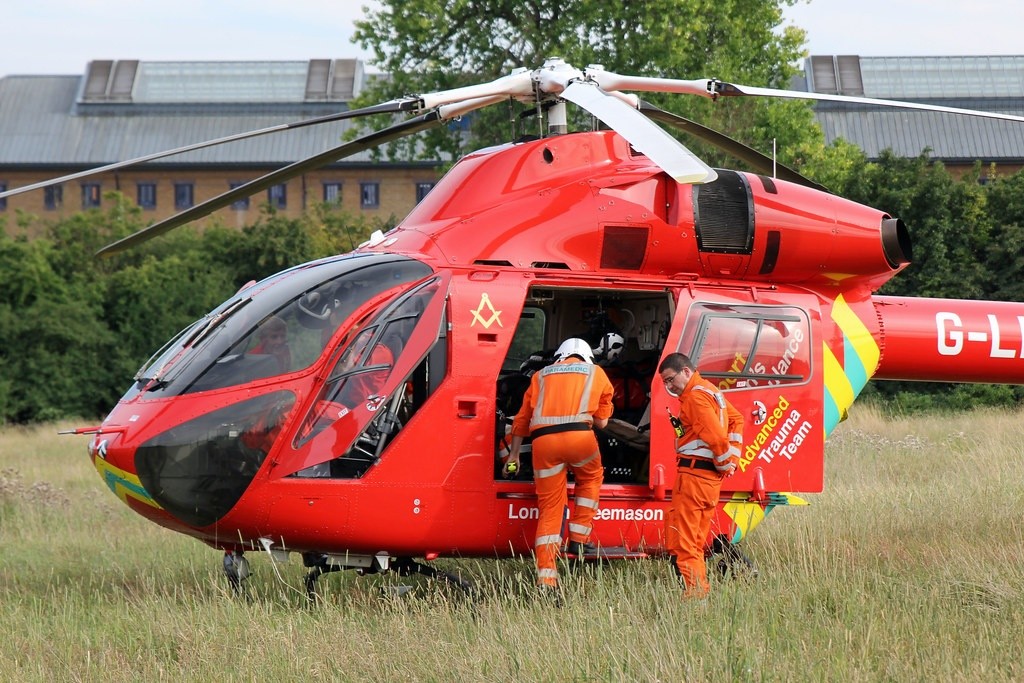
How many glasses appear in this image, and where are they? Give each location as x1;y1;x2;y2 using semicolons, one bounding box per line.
664;371;681;385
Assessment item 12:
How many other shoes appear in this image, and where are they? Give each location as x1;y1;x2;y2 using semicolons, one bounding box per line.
538;584;561;608
568;541;595;555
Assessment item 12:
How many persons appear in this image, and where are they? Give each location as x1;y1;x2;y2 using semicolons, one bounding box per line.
500;333;645;463
223;314;291;569
658;353;744;608
242;304;394;458
502;338;615;604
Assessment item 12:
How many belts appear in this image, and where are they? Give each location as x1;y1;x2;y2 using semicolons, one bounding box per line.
678;458;718;471
531;422;590;441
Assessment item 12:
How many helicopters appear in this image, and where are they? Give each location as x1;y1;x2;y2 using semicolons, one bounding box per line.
0;56;1024;618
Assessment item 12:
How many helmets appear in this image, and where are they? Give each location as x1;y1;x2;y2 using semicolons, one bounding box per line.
553;338;594;365
593;333;624;359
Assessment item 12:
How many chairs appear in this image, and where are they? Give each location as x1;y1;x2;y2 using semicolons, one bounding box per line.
604;404;650;449
